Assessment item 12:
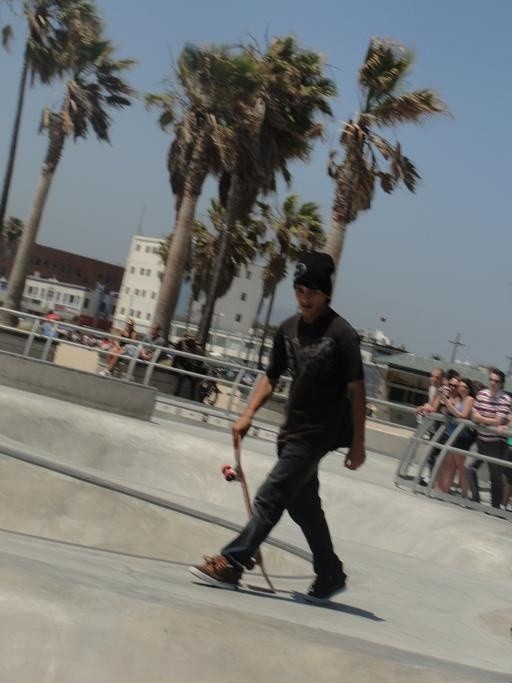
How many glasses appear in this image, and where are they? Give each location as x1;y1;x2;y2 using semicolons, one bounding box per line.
448;382;457;387
488;377;501;383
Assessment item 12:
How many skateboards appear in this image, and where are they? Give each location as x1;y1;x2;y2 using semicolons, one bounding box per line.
223;427;275;594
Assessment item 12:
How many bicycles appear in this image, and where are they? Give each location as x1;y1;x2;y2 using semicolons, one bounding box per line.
197;367;218;407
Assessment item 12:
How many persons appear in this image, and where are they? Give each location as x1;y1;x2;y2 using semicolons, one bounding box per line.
98;322;165;377
413;361;512;512
104;317;138;364
186;250;371;604
173;329;197;367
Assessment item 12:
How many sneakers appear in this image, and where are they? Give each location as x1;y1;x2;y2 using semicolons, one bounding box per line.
303;576;349;604
189;555;242;591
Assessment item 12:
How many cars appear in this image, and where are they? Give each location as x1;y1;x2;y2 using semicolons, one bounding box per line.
208;349;387;417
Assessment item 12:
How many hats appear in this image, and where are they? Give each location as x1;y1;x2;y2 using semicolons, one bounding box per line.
293;252;334;296
